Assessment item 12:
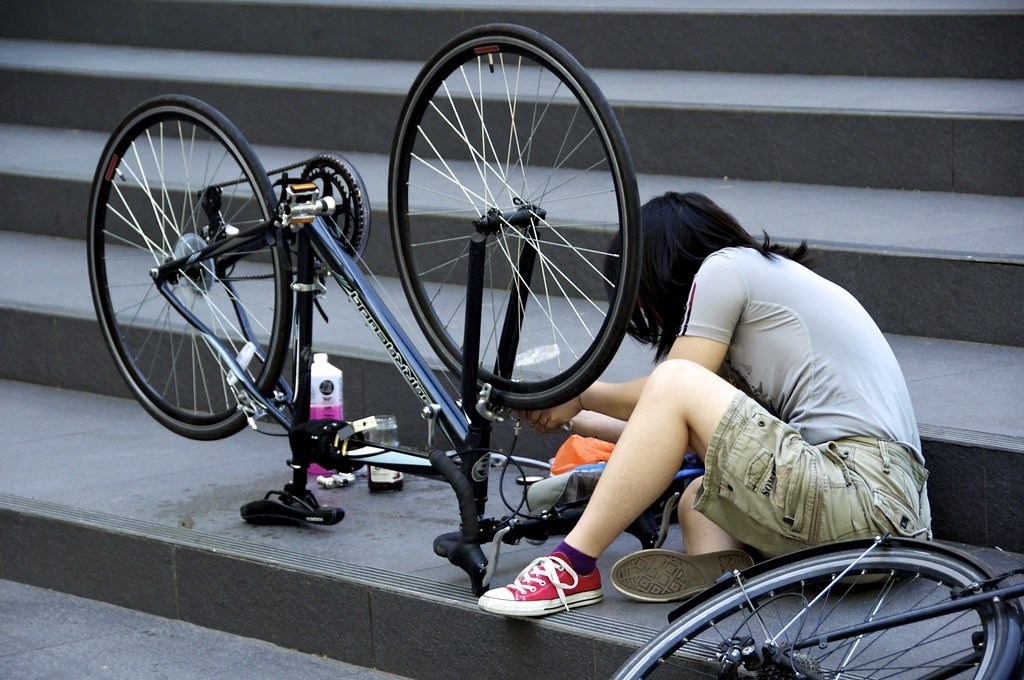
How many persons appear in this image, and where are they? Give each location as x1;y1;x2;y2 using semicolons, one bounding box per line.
478;190;931;617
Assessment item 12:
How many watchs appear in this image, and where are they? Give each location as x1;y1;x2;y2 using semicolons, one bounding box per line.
563;419;574;432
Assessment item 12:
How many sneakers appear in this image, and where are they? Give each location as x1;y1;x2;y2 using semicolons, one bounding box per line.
610;548;754;602
477;552;604;617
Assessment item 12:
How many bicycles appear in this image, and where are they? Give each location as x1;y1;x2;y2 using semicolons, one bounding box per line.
609;529;1024;680
84;22;682;592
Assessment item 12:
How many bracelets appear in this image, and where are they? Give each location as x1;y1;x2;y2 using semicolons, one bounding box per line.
578;396;590;411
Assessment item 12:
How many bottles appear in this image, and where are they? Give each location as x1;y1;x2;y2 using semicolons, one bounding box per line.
306;353;343;477
365;414;403;491
